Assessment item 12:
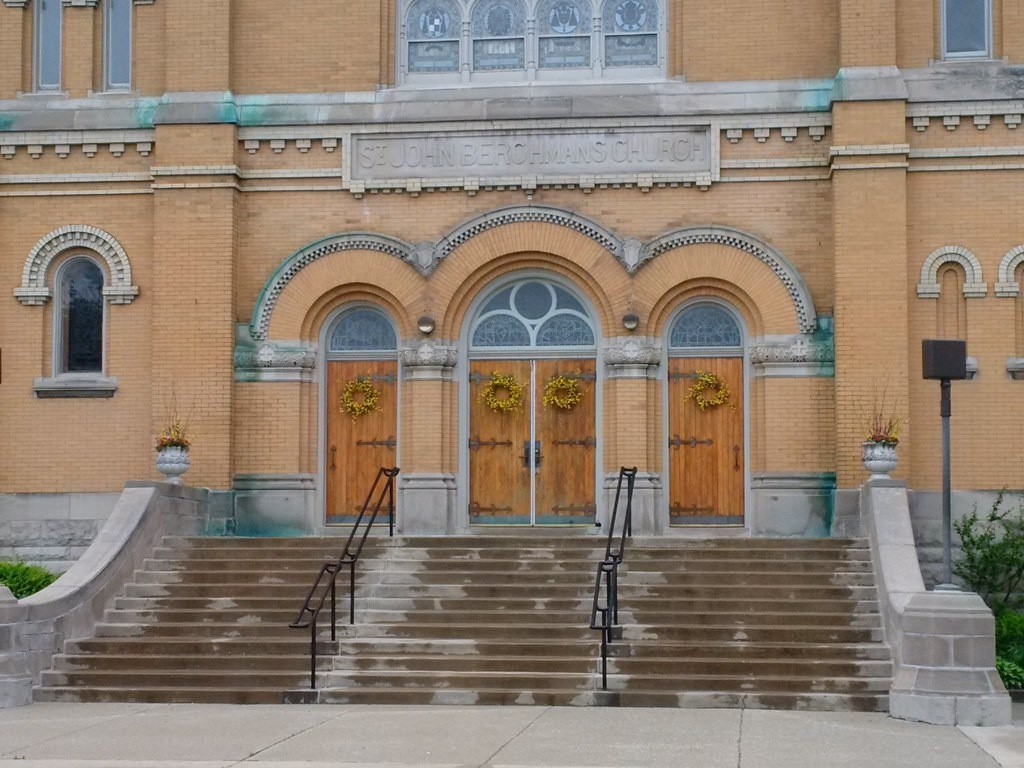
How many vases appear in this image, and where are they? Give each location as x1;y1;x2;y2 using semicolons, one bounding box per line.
860;440;899;480
155;445;190;485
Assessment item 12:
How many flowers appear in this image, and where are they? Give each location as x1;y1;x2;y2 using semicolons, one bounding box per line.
862;412;901;445
337;368;383;423
537;366;590;413
155;418;193;452
688;371;738;416
477;369;529;416
